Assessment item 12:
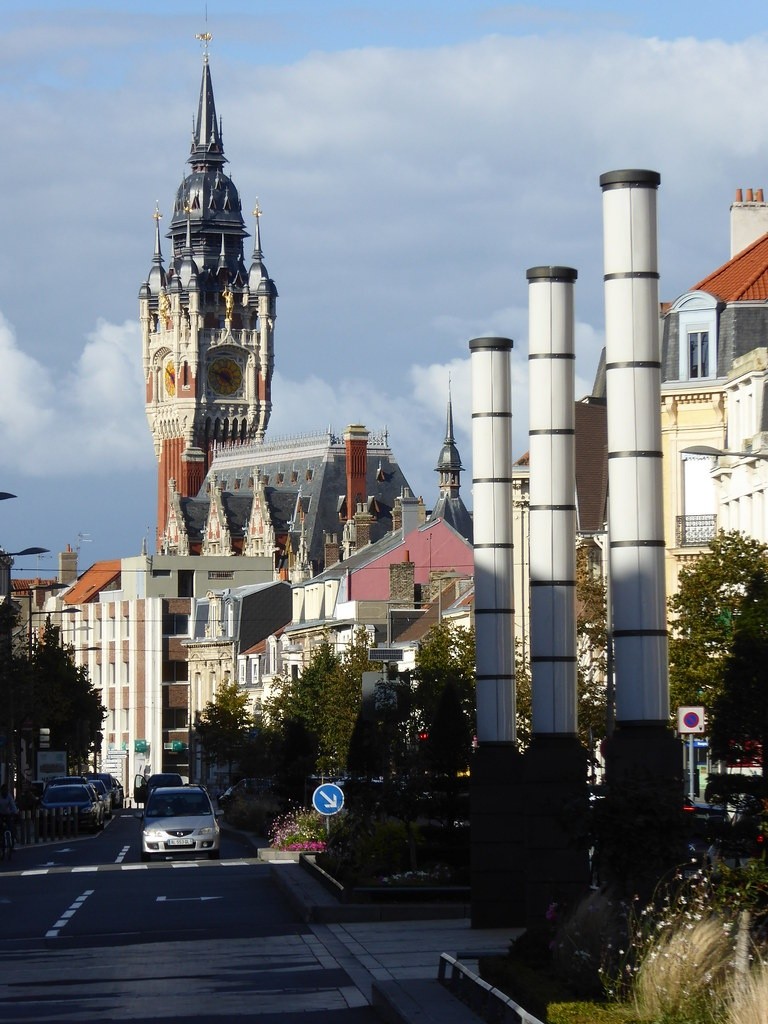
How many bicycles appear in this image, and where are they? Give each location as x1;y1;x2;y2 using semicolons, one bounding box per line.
0;812;18;862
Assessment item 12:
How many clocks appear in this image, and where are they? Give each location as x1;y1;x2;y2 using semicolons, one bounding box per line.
164;360;175;397
207;356;243;398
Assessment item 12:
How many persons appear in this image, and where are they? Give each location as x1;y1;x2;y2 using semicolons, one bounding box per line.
25;785;41;823
0;784;18;815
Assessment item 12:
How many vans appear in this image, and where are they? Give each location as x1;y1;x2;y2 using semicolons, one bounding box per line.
134;773;189;809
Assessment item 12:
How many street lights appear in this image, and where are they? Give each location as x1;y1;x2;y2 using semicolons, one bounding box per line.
12;582;70;781
51;634;102;775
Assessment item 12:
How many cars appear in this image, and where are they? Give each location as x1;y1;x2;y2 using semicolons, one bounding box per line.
133;783;225;862
40;773;124;820
218;774;433;812
37;784;100;836
